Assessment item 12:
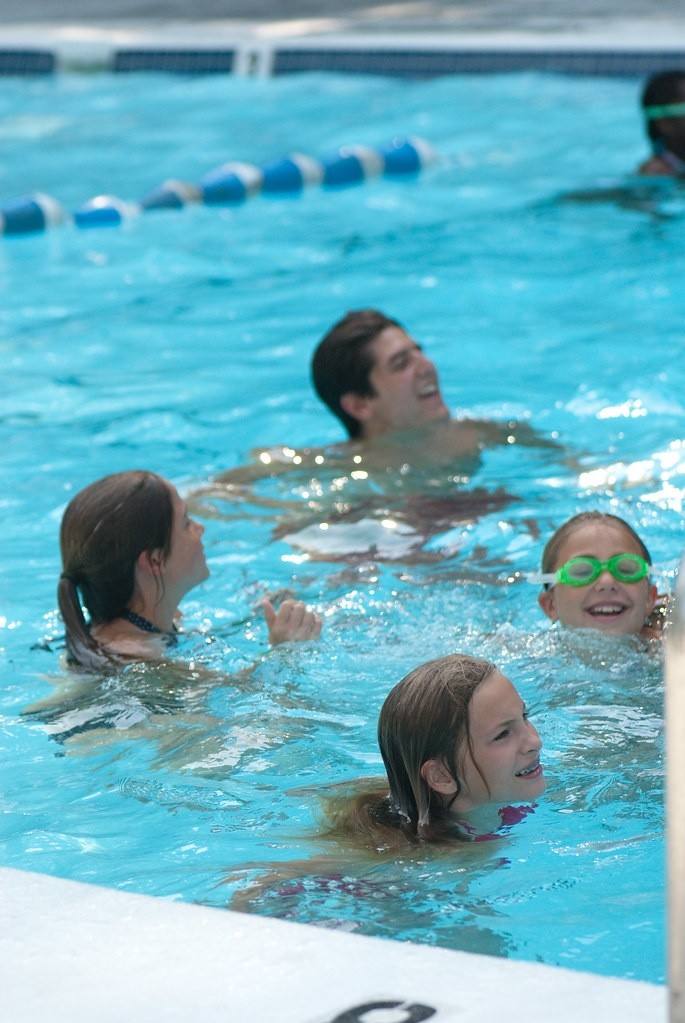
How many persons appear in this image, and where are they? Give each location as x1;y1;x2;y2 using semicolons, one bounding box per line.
633;72;685;181
57;470;322;696
198;310;567;494
237;654;545;930
532;512;660;654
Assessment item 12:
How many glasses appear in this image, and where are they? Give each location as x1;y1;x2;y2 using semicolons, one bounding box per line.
546;554;653;588
